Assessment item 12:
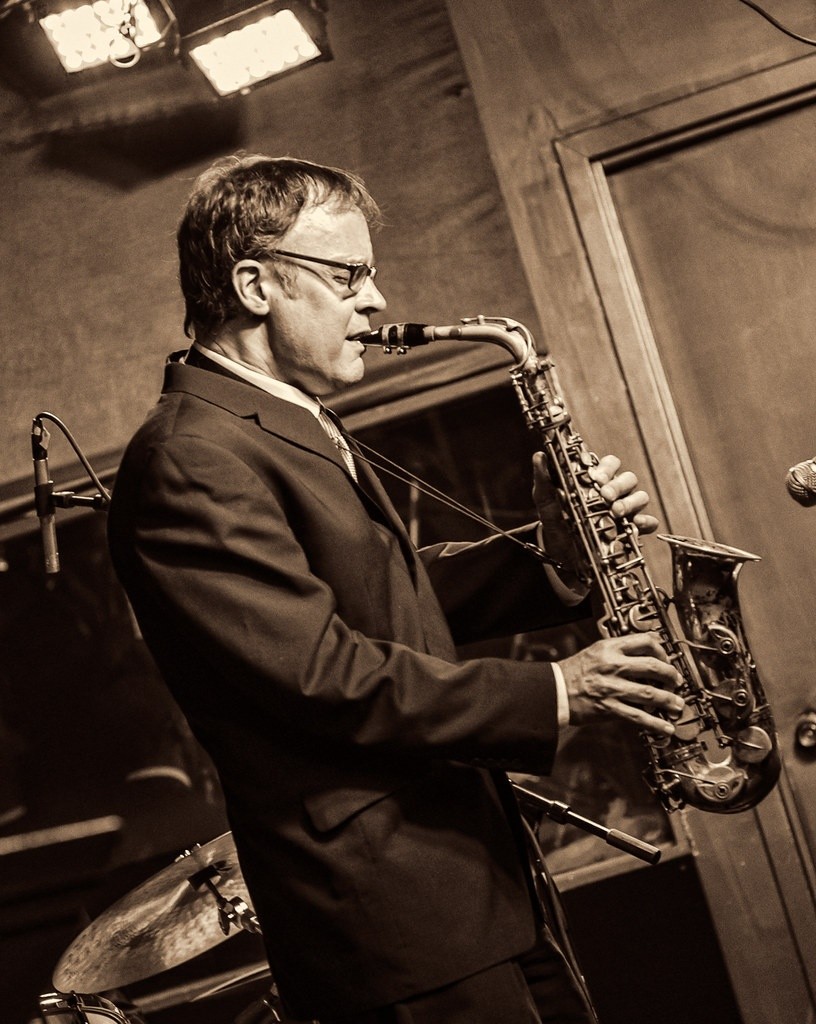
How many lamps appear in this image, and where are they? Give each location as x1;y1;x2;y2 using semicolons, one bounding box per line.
175;0;336;103
24;1;176;75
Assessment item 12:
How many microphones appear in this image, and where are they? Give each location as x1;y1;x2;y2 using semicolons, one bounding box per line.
785;457;816;508
31;418;60;573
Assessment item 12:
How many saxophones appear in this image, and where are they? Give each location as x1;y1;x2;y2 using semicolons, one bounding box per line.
362;313;783;813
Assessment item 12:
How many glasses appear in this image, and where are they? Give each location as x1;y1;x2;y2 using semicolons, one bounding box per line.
252;246;380;293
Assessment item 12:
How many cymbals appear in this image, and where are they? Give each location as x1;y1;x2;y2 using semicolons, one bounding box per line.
53;830;256;996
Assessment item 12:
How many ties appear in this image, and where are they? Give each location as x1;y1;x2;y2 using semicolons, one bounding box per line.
319;411;361;486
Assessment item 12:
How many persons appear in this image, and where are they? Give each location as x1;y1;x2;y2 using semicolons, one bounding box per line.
109;156;685;1023
